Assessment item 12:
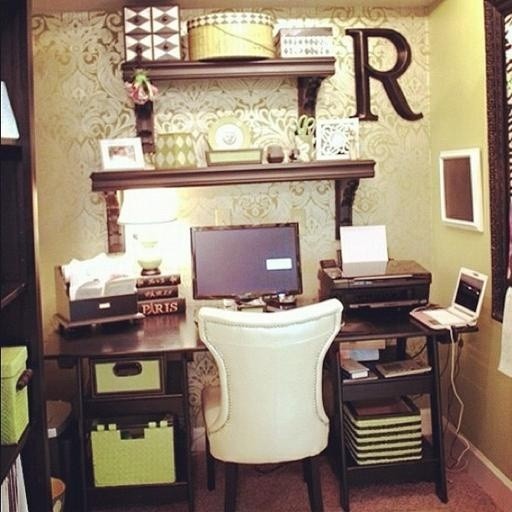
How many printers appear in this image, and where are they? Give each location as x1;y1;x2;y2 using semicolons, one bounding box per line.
318;249;433;323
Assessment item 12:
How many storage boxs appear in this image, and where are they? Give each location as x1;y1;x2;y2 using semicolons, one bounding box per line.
86;412;177;488
0;345;30;446
341;395;424;467
274;27;336;59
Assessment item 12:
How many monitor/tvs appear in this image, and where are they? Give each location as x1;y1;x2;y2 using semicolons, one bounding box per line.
190;221;303;310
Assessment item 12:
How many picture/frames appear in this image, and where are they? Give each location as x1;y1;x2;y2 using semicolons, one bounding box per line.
99;137;147;173
439;147;484;234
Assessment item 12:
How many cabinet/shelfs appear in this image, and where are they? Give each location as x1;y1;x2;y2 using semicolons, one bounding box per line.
0;0;53;512
339;335;449;512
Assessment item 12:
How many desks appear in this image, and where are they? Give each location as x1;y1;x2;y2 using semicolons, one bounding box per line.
40;299;479;512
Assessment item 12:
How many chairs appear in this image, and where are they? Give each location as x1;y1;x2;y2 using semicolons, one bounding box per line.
197;297;344;512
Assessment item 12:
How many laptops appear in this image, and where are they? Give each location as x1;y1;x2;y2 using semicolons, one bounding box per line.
409;268;488;330
